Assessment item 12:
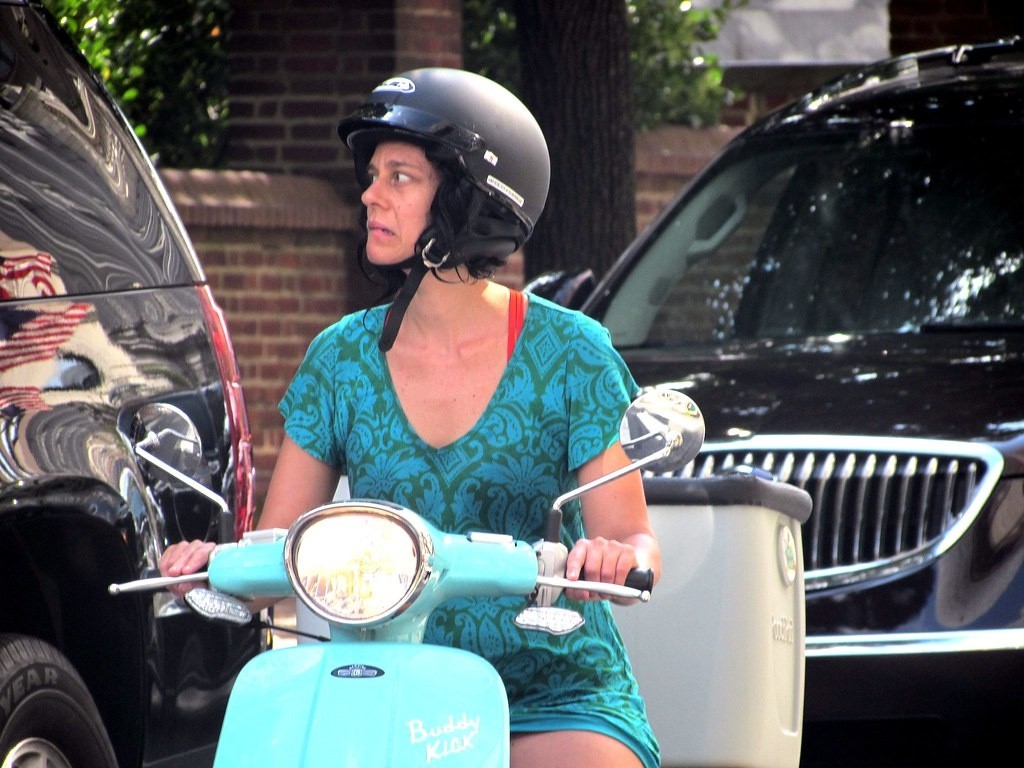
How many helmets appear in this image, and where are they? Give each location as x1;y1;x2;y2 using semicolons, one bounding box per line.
337;68;552;234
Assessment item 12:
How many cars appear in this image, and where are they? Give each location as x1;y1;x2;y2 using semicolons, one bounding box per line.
0;3;272;768
567;29;1024;768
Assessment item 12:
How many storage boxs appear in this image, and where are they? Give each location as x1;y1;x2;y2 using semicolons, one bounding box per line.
612;474;813;768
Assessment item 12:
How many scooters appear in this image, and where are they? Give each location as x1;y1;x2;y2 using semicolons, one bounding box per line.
109;383;812;768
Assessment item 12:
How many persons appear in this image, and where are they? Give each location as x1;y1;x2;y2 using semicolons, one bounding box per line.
150;65;664;768
